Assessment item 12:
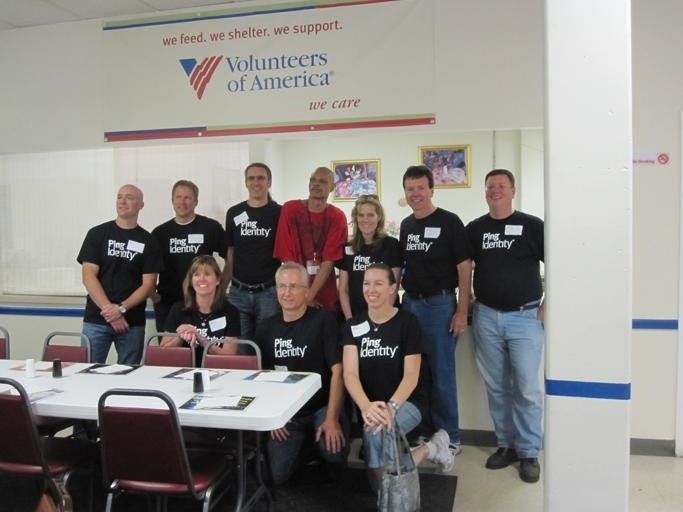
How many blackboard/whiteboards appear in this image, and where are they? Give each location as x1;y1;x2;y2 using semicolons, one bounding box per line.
0;142;250;297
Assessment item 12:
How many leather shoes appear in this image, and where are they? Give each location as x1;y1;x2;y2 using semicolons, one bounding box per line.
517;456;541;483
485;445;521;469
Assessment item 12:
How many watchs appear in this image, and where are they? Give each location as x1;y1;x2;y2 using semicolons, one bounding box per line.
117;302;127;315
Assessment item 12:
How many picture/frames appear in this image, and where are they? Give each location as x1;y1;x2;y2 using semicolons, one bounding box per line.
417;144;471;191
329;159;381;203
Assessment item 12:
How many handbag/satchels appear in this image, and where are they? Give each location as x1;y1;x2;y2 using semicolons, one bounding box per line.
375;463;422;512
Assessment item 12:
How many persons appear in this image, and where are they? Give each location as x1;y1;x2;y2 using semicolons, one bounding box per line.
150;179;226;346
71;184;156;441
272;165;349;323
157;254;243;369
465;168;544;484
335;263;456;495
396;165;474;459
245;261;350;493
220;162;285;356
330;193;404;323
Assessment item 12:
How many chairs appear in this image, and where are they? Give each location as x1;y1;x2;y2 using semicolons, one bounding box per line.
32;330;90;438
95;388;238;512
181;336;273;511
0;324;10;359
141;331;196;366
0;372;101;512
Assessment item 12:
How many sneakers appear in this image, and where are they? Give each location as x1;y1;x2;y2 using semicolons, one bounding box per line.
408;435;430;452
448;440;463;457
427;427;456;473
317;460;340;489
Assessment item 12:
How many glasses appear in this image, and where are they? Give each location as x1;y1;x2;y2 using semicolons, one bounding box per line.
275;282;309;290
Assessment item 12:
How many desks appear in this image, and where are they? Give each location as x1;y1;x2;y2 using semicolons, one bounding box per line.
0;359;322;512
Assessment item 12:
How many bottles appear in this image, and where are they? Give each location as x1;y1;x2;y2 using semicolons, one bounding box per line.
25;358;35;378
192;370;209;393
51;359;61;378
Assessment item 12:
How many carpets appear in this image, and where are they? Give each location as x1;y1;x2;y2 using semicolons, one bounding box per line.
251;463;459;512
